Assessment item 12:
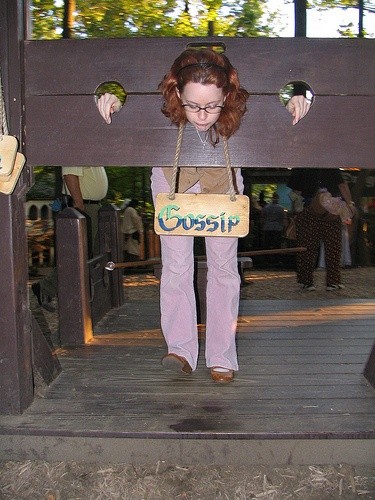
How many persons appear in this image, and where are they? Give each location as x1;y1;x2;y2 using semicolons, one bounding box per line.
287;166;355;291
31;166;109;313
96;46;311;384
261;191;288;263
318;195;351;269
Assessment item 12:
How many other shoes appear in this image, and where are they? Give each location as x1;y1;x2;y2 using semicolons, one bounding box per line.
326;283;345;292
209;366;235;384
161;352;193;378
303;284;316;291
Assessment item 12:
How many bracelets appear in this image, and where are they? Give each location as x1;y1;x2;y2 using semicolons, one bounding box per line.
350;201;354;204
349;204;353;206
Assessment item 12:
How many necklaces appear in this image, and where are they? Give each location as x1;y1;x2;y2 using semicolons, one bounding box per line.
197;131;209;147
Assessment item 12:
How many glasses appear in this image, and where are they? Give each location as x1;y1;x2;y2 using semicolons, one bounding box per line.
180;100;225;114
